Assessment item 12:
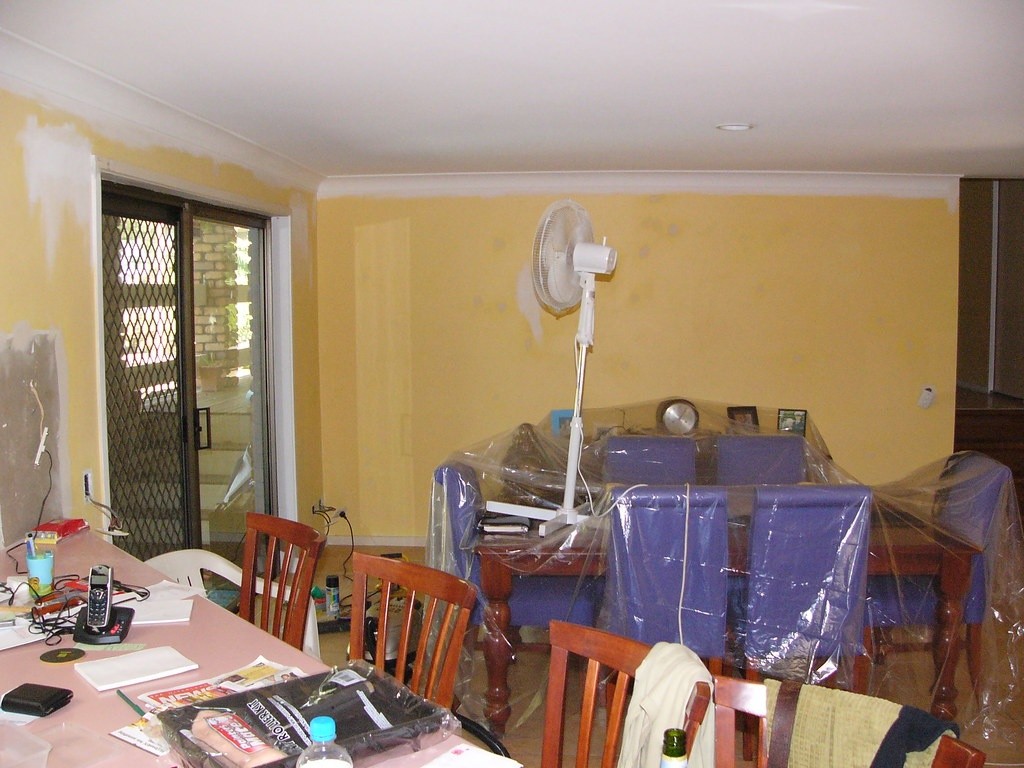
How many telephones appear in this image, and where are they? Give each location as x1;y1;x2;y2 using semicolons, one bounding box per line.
71;562;136;644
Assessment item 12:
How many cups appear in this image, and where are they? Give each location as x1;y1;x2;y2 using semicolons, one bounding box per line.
25;552;55;600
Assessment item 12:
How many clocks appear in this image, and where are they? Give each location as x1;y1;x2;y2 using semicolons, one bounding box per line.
656;397;699;435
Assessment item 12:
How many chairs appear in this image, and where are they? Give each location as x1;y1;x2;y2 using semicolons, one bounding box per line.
714;676;986;768
541;619;711;768
350;551;478;710
141;549;321;659
848;450;1012;717
239;511;327;652
599;434;873;762
434;449;605;722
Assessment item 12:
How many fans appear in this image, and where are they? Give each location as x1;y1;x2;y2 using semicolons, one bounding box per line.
486;198;611;538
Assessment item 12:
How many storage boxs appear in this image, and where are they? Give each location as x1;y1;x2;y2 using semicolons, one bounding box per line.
34;519;84;535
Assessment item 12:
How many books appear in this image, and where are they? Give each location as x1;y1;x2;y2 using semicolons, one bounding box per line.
161;662;447;768
113;601;194;624
478;516;531;533
74;643;199;692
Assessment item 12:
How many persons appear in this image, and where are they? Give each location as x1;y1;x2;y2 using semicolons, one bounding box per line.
561;419;570;438
781;411;804;436
281;674;297;683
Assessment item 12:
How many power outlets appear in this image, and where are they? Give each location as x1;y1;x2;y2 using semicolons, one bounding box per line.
324;508;346;527
96;528;129;537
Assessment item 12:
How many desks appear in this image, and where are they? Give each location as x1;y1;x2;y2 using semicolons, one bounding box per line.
0;531;523;768
472;495;981;739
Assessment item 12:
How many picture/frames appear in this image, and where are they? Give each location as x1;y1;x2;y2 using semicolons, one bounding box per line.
777;409;807;437
550;409;574;437
727;406;759;434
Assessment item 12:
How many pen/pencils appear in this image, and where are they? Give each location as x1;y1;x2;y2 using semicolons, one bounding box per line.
25;532;36;560
114;687;144;718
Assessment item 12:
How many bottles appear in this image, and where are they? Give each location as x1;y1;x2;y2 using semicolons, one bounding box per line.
325;575;340;621
659;727;690;768
295;715;355;767
